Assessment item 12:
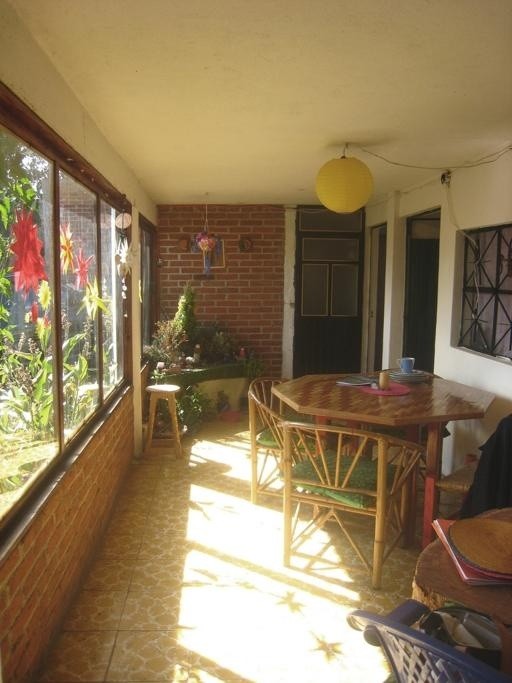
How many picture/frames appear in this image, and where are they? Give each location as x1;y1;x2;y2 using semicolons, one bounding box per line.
203;239;226;269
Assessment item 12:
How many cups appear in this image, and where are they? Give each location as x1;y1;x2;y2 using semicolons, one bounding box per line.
395;358;415;375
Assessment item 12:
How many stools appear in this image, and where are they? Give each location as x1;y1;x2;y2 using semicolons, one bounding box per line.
143;384;182;459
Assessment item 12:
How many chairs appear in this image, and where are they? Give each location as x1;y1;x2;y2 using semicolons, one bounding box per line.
250;378;361;528
434;413;511;542
346;599;511;682
277;420;424;591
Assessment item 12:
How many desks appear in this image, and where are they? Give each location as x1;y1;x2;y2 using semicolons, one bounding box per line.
412;536;511;627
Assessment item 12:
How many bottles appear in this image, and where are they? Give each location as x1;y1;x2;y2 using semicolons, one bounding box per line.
379;373;388;389
239;347;245;358
194;344;202;364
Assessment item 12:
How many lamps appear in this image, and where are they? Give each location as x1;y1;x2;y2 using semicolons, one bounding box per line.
315;143;373;214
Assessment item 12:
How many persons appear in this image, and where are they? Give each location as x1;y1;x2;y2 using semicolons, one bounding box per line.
460;411;512;518
